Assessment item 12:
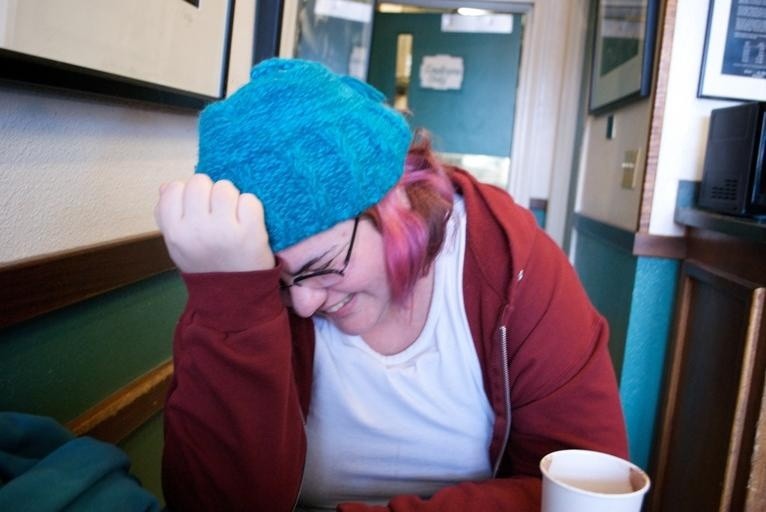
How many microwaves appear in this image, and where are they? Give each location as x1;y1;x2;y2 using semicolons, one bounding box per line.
696;99;766;221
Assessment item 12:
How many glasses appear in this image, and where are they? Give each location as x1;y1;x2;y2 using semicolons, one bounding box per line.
280;215;359;309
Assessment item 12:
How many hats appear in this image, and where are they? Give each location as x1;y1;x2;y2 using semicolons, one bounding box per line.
193;55;414;258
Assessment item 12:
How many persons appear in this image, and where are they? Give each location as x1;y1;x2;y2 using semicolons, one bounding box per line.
154;57;630;512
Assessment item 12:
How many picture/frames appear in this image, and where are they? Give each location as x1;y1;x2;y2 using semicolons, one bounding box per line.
587;0;657;115
697;0;766;105
0;1;236;107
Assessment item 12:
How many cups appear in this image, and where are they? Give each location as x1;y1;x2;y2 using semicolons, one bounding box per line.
539;447;653;512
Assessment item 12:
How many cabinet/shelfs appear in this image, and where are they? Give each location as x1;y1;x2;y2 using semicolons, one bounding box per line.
639;209;765;511
